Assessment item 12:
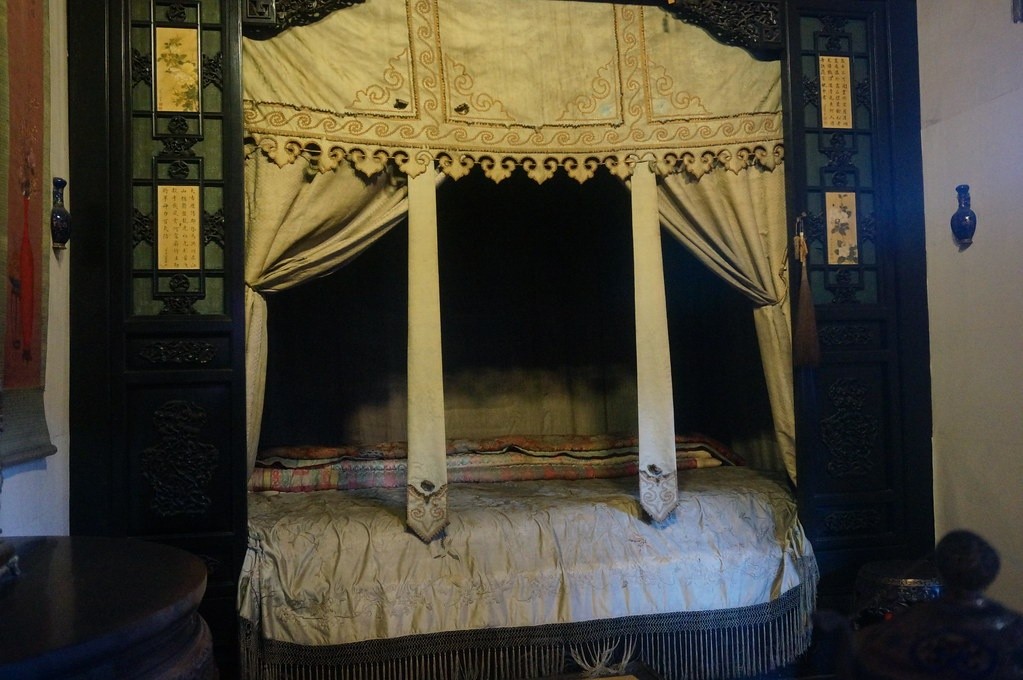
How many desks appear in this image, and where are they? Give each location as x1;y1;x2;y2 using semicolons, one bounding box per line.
0;536;215;680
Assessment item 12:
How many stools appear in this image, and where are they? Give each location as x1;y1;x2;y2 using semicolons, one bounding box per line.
849;557;943;623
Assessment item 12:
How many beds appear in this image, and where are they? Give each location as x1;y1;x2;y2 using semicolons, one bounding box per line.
229;363;821;680
